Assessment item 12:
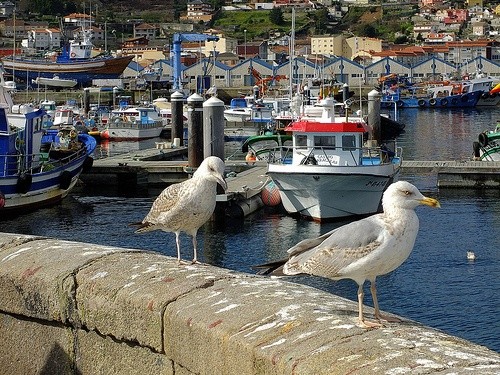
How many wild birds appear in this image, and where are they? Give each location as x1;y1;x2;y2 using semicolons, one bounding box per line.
250;180;442;330
132;155;228;267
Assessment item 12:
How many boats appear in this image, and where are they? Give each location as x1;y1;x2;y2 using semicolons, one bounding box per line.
0;7;500;161
266;95;403;225
0;102;97;216
473;122;500;161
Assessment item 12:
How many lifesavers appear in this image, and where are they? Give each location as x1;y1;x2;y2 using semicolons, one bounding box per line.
440;97;448;105
15;174;32;194
428;98;436;105
396;100;404;107
59;170;71;189
452;97;459;105
473;142;480;157
461;94;469;103
479;133;487;147
83;157;93;173
418;98;426;106
